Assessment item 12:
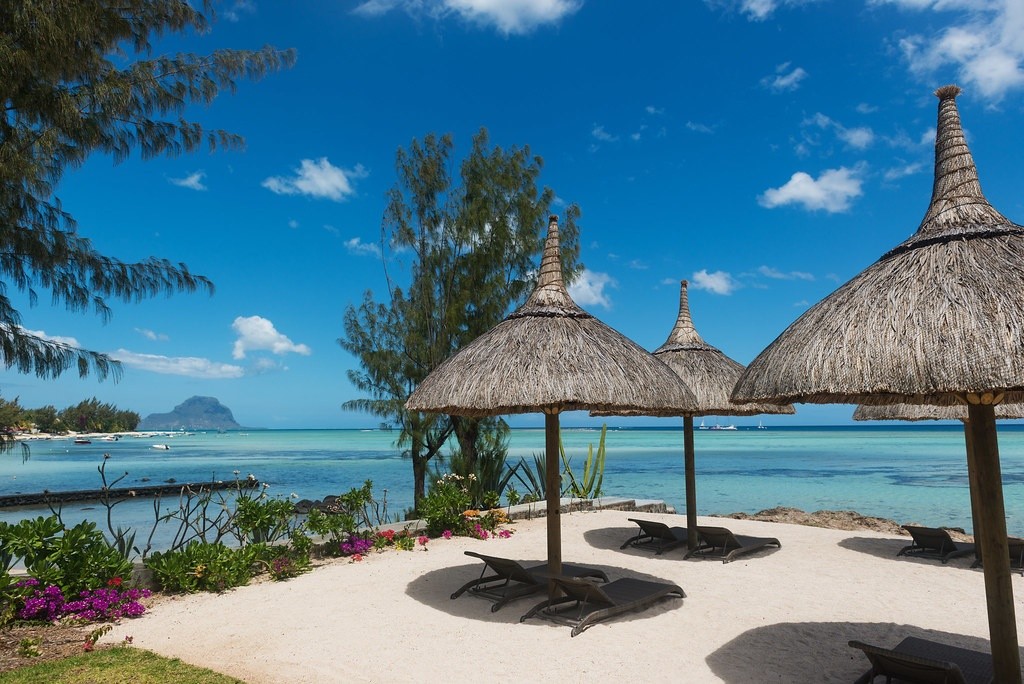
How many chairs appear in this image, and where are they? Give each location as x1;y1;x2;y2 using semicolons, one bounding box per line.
450;550;610;613
897;524;976;564
520;576;687;637
620;518;688;555
845;634;995;684
682;525;781;564
970;537;1024;579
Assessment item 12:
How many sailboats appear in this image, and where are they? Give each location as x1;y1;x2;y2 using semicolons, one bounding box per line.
699;416;767;431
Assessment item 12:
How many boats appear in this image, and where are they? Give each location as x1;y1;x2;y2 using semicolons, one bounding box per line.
73;436;91;444
153;444;170;450
105;435;118;441
